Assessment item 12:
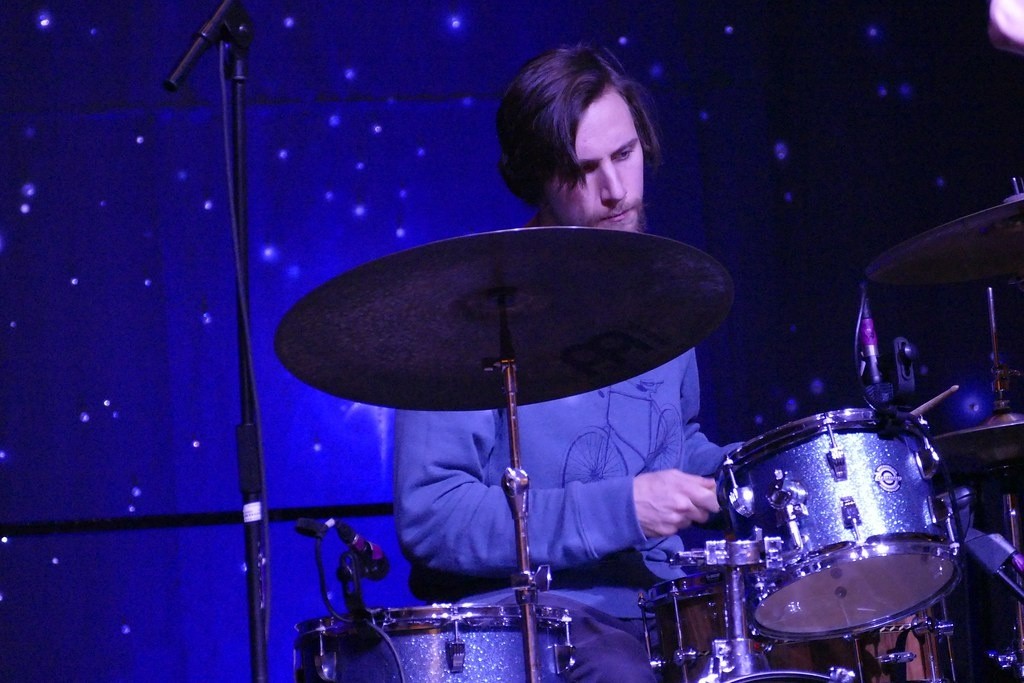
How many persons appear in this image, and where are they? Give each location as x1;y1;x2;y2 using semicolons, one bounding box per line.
397;41;752;683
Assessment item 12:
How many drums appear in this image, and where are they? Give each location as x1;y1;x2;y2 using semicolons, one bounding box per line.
713;405;965;641
292;599;573;683
642;567;956;683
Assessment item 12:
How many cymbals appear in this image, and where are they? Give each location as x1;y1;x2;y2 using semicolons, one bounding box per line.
863;196;1024;287
925;413;1024;463
274;222;737;412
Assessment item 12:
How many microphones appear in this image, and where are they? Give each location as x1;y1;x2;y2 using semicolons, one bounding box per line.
862;295;894;406
335;522;390;582
